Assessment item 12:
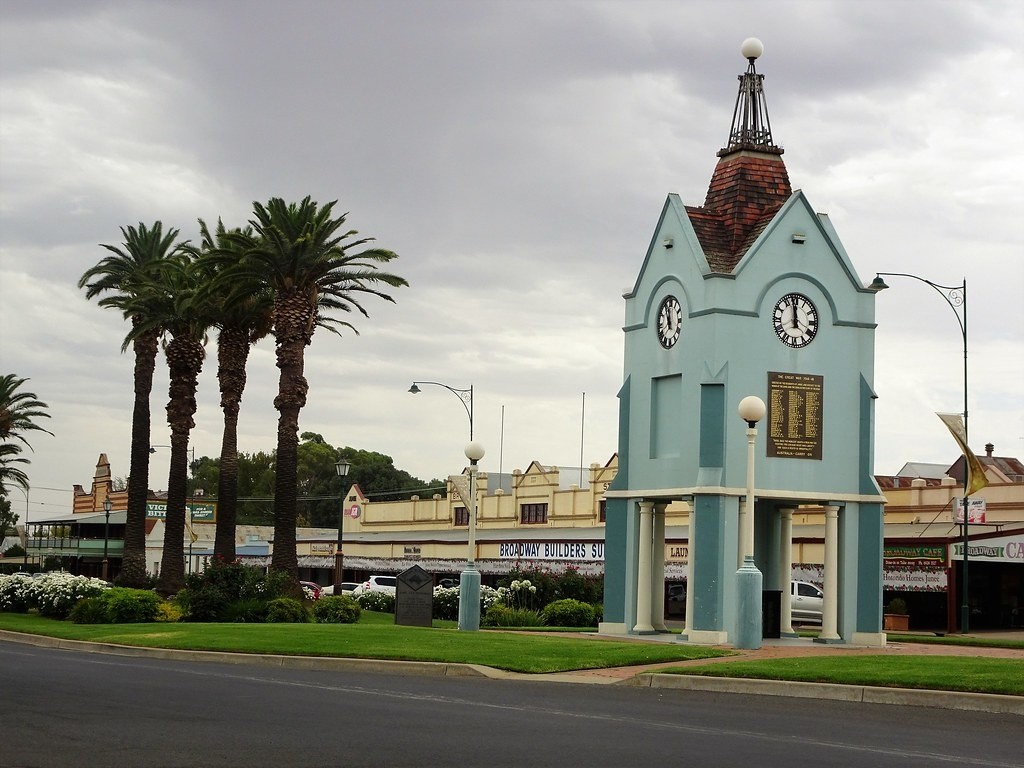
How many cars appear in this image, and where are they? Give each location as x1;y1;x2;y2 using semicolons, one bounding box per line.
13;573;33;582
787;581;823;625
323;582;362;595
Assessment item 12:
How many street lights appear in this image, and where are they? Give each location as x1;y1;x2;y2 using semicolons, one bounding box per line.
150;445;193;577
870;273;971;633
101;496;112;581
734;397;765;649
408;381;474;502
459;440;487;631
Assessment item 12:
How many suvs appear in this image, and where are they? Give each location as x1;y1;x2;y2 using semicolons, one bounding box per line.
363;576;397;597
300;580;323;600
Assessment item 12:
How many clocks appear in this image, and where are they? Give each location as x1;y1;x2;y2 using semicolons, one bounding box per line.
772;294;817;348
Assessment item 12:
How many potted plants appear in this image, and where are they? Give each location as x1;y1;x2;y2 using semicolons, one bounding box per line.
656;296;681;350
